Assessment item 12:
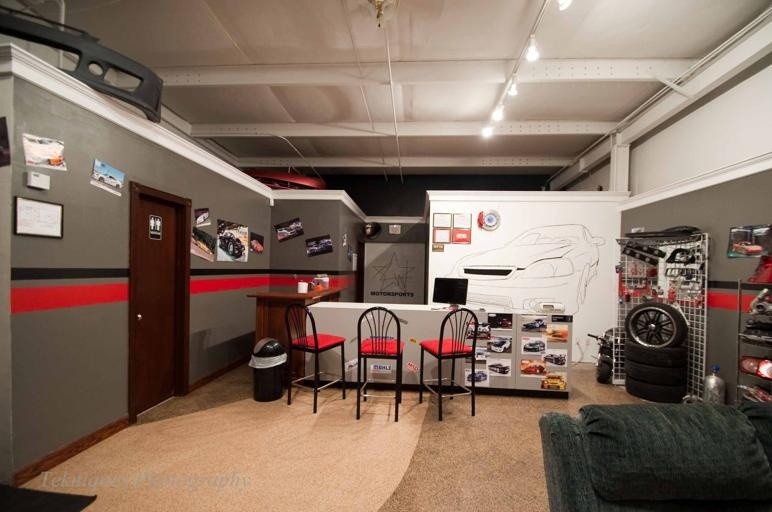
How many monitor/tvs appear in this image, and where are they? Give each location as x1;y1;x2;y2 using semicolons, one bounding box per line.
433;278;468;306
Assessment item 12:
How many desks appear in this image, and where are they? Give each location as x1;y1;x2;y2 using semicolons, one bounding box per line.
245;285;340;385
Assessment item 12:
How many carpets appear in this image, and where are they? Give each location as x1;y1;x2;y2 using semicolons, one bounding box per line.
0;485;96;512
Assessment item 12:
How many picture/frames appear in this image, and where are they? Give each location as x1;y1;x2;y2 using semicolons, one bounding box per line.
14;195;64;239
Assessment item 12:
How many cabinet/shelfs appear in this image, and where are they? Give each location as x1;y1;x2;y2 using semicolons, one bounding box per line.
736;278;772;404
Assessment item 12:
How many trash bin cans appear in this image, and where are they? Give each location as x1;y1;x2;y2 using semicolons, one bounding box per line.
251;337;288;402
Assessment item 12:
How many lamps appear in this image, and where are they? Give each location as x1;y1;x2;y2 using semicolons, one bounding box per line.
480;0;571;140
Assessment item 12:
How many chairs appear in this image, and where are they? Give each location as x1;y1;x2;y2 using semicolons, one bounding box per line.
285;303;346;413
419;308;479;421
357;306;405;420
539;400;771;511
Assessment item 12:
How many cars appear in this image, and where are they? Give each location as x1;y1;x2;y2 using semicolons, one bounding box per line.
307;238;333;257
732;240;763;257
278;221;303;238
197;212;209;225
45;153;64;167
93;172;122;191
521;319;568;391
447;223;607;316
196;239;214;255
218;231;245;258
250;238;263;254
463;315;512;383
